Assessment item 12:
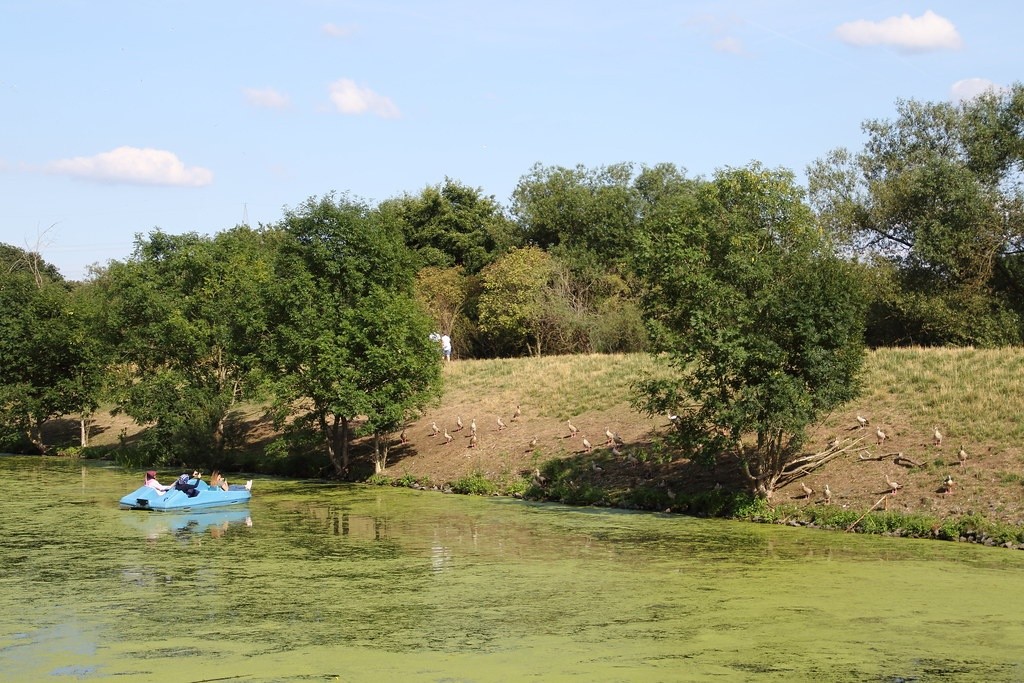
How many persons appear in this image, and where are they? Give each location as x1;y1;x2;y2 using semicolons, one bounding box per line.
143;470;175;497
176;471;201;497
430;331;452;361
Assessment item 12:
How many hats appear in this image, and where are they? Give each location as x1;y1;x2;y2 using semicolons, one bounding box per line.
147;471;156;479
178;474;189;484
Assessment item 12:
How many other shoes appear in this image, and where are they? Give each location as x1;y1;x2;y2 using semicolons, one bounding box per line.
245;479;252;491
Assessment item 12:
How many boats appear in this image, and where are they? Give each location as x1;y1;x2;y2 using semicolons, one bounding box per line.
118;476;253;512
